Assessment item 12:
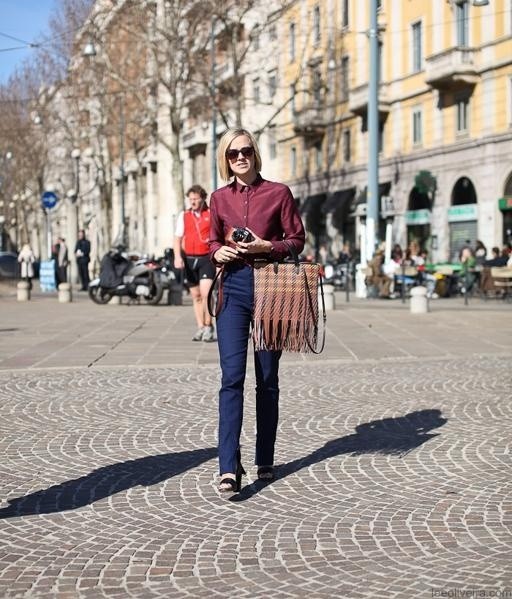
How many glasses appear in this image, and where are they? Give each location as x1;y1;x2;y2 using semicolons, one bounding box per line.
225;146;255;160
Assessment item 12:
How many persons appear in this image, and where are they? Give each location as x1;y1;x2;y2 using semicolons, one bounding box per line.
173;185;217;343
207;129;306;492
17;243;35;290
299;239;512;301
57;237;68;282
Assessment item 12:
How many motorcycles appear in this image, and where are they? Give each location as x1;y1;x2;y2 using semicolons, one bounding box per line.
88;248;176;305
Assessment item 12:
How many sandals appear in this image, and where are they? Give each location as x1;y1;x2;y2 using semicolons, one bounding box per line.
217;478;242;493
257;467;274;482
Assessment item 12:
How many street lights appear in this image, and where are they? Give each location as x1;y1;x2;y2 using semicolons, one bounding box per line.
66;148;100;287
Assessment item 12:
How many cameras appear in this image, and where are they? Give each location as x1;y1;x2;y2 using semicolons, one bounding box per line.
224;226;251;248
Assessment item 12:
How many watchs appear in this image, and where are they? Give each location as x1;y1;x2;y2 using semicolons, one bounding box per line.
75;229;91;292
270;242;276;255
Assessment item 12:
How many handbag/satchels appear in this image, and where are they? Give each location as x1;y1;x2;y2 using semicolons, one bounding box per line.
249;240;326;355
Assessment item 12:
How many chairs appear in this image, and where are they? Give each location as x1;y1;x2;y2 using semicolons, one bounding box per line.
477;266;510;302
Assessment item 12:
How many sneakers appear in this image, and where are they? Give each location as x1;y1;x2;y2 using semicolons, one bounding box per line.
192;328;204;341
202;326;214;341
